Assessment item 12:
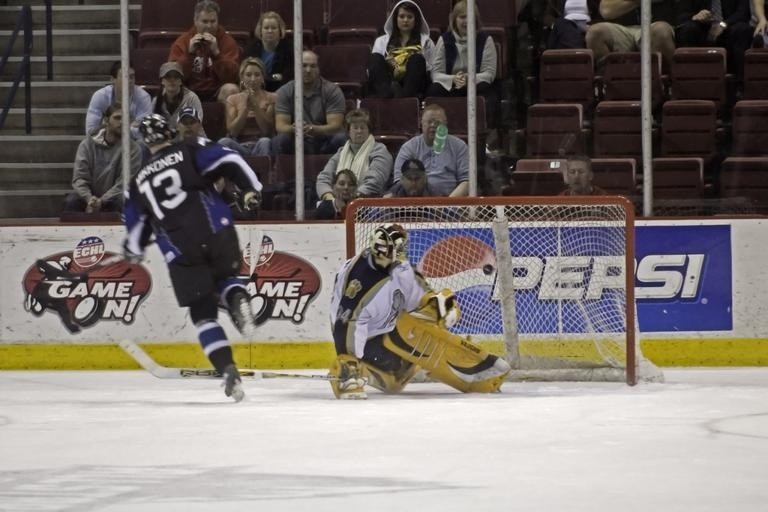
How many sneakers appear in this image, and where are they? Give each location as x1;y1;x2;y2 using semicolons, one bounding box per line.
232;291;249;333
223;364;241;397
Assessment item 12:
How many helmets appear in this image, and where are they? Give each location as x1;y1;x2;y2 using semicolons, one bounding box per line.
370;223;407;260
138;113;176;146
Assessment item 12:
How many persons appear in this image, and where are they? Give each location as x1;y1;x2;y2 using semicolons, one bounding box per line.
516;0;768;91
62;1;345;219
545;154;613;217
124;111;266;398
330;222;512;399
315;103;472;218
363;0;500;159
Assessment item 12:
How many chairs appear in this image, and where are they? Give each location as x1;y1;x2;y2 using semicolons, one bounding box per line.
133;0;768;214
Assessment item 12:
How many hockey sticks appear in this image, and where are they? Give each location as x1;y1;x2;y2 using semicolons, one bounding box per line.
36;199;239;277
120;338;346;381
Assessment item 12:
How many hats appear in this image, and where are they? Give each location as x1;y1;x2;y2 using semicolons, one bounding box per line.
401;158;426;176
159;62;184;78
177;106;201;124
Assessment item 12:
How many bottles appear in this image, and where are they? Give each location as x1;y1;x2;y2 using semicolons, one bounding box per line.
432;125;448;154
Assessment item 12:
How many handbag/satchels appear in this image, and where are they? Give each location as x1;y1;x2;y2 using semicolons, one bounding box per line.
387;45;425;71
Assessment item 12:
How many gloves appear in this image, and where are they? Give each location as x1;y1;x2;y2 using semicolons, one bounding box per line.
238;187;263;212
